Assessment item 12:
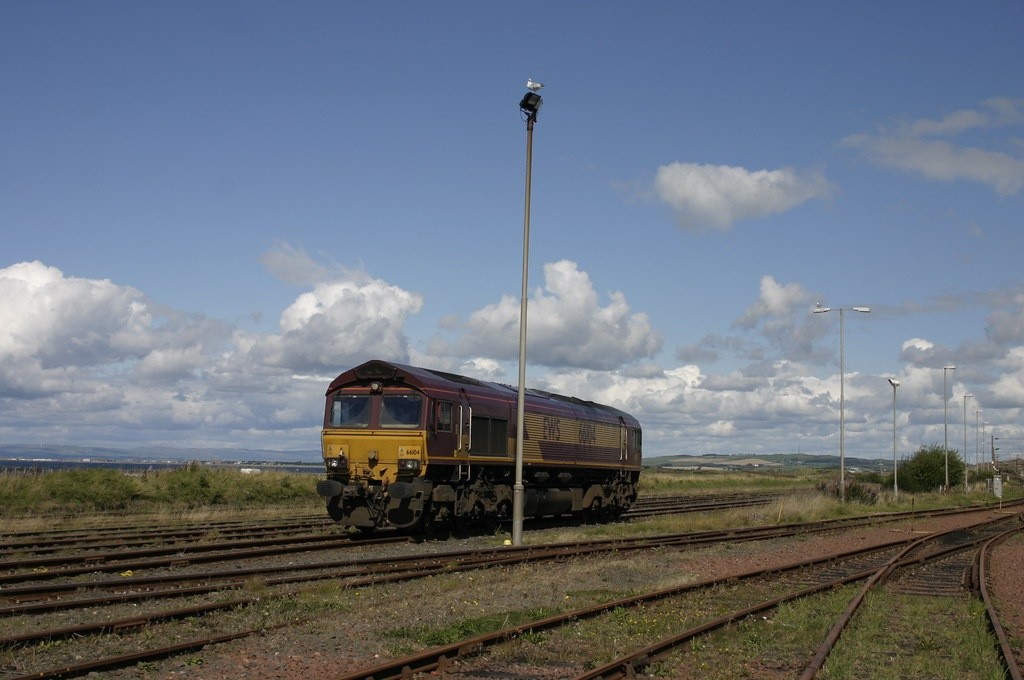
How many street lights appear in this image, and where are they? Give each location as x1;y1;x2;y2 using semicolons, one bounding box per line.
943;366;956;493
812;301;871;506
963;394;974;489
507;79;544;547
981;421;990;472
888;378;901;499
976;410;983;477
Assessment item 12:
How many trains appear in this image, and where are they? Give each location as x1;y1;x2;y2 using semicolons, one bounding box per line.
315;360;642;538
